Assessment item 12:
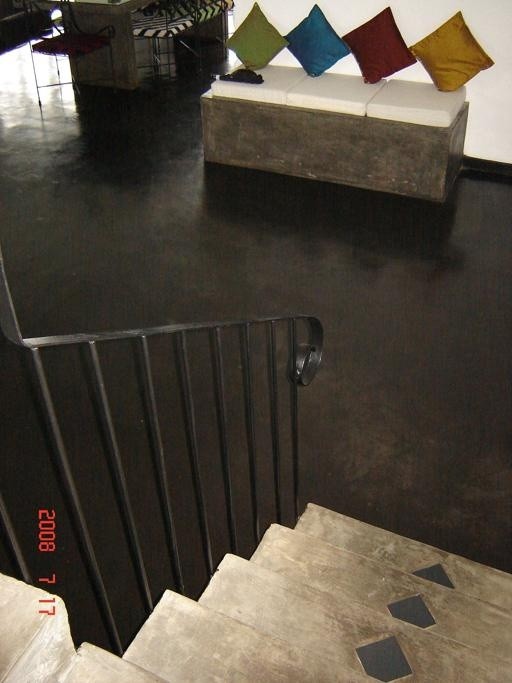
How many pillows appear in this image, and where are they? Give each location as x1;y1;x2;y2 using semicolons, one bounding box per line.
223;2;496;93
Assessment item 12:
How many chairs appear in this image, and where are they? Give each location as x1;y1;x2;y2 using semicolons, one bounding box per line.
21;0;117;107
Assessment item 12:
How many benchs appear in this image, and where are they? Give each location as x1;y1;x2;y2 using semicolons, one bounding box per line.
197;65;471;218
